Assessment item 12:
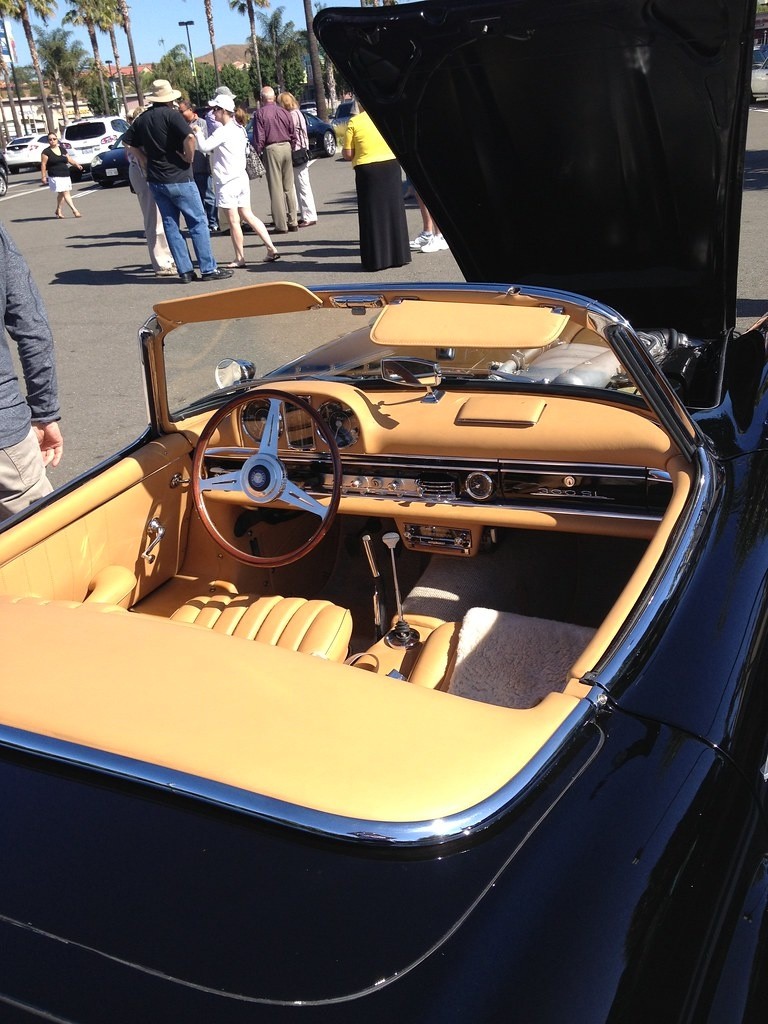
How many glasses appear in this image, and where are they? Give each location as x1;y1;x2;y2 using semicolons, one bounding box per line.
179;107;190;114
48;137;57;141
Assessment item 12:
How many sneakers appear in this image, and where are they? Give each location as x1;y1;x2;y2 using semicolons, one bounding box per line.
180;270;198;285
201;266;234;281
420;234;449;253
407;232;434;251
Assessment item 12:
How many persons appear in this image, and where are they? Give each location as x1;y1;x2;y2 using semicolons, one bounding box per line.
126;86;282;269
252;86;318;234
40;132;84;218
0;218;63;522
121;78;233;283
407;188;449;252
341;98;413;272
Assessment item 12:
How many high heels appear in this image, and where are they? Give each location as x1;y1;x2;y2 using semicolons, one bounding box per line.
72;209;82;218
55;210;65;219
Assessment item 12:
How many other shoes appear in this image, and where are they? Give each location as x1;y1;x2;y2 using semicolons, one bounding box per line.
404;191;416;200
288;226;298;232
268;229;289;234
155;261;179;275
208;224;220;232
263;252;280;262
228;258;246;268
240;220;256;232
297;219;317;228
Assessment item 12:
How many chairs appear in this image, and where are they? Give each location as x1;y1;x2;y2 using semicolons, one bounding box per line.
406;607;600;710
167;593;352;664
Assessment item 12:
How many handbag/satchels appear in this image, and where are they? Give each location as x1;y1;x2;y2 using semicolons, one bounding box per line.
236;124;267;181
291;148;310;167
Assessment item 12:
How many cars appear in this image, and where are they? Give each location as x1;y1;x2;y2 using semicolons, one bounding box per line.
328;99;363;139
88;133;136;188
240;109;339;168
299;102;319;117
0;153;11;195
4;133;60;174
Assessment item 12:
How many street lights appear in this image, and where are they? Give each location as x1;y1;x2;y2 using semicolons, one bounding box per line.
177;21;212;108
104;59;113;76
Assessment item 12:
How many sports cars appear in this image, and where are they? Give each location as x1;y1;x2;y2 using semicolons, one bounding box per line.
0;0;768;1022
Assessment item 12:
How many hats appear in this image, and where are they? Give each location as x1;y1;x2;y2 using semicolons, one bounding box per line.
208;94;235;112
144;78;182;103
214;86;237;100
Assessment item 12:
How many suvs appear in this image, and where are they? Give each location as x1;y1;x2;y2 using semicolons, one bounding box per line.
59;117;135;174
